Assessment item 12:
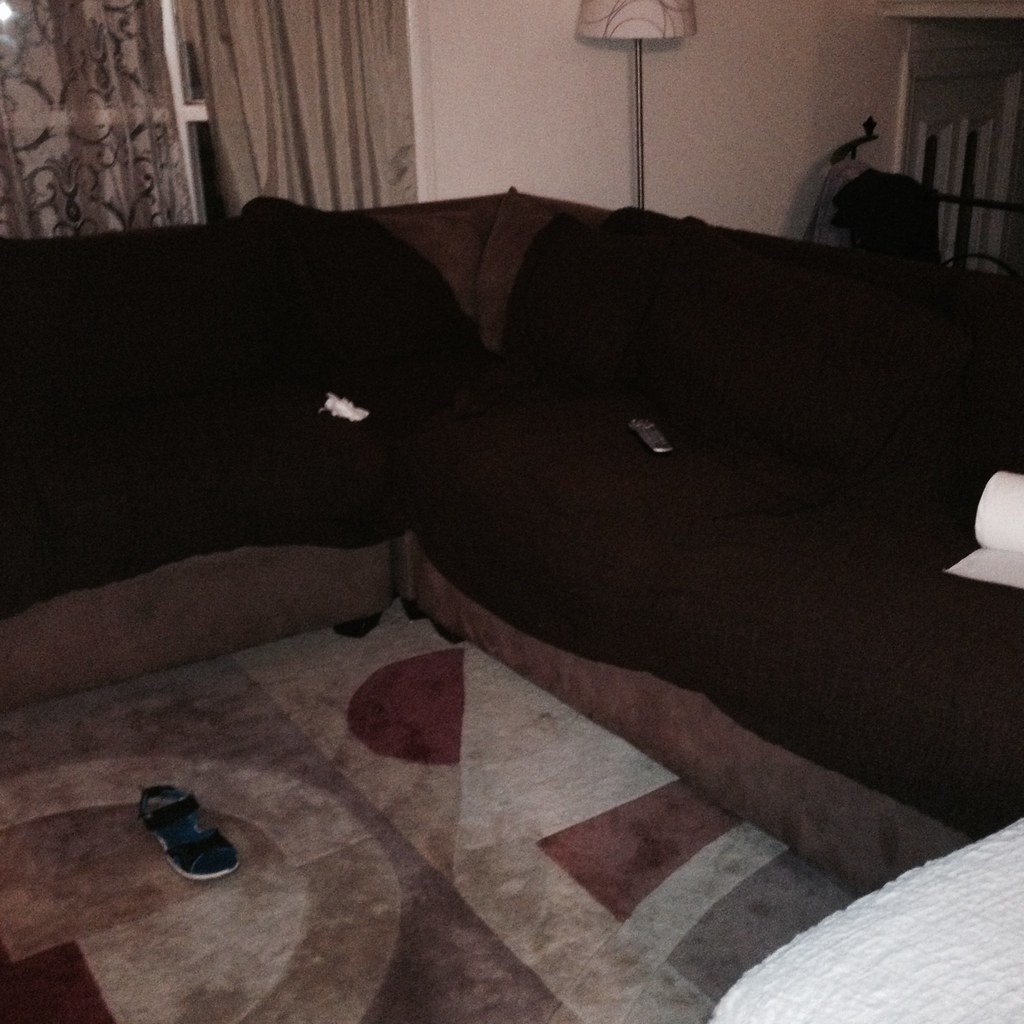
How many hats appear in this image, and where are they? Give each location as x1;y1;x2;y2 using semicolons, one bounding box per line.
345;647;466;764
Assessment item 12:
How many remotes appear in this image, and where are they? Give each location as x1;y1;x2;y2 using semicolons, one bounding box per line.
628;418;674;457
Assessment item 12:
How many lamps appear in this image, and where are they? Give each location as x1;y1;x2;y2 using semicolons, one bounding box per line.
573;0;697;212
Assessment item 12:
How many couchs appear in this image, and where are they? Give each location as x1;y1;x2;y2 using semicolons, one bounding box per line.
0;188;1024;888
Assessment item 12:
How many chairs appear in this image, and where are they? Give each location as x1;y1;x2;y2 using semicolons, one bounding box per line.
800;158;873;250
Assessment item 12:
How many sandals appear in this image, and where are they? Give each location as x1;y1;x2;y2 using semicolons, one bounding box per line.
136;780;244;879
